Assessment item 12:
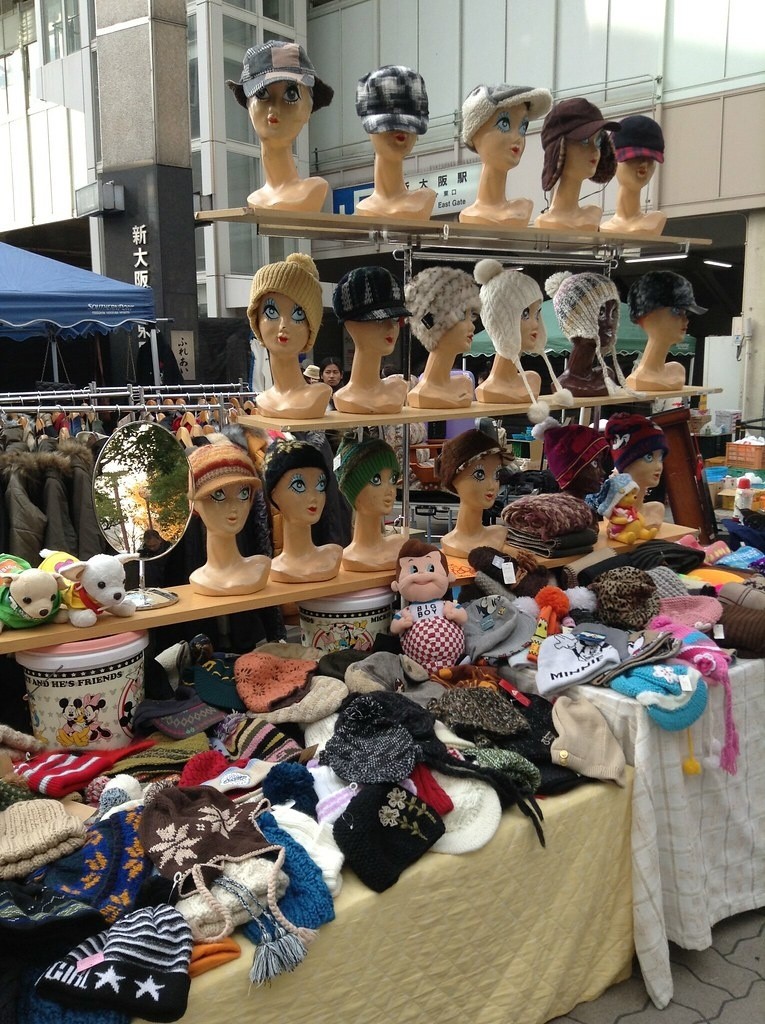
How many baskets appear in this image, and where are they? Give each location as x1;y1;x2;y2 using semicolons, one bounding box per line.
726;441;764;471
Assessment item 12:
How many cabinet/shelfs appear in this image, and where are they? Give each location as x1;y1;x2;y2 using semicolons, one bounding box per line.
1;206;712;653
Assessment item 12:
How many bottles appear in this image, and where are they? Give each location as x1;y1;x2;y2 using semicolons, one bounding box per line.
733;477;753;522
720;425;725;434
705;426;711;435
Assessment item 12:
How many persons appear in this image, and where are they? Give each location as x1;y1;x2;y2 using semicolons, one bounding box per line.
333;264;408;415
625;270;709;390
440;428;509;561
224;39;334;214
458;81;554;228
136;529;173;558
355;62;437;222
544;270;646;399
262;440;343;584
245;252;332;421
184;443;273;597
474;257;574;421
334;435;410;573
403;266;482;409
322;356;343;391
600;115;668;237
530;417;612;502
534;98;623;236
608;411;670;529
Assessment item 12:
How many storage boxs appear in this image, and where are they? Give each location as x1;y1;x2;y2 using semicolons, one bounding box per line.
726;442;765;470
717;489;765;512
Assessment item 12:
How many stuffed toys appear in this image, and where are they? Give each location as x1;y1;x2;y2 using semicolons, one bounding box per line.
389;540;468;678
38;546;139;628
585;462;658;543
0;551;68;634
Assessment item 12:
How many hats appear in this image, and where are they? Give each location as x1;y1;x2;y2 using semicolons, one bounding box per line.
544;270;646;400
540;97;622;192
628;270;707;322
436;429;515;497
608;115;666;162
462;83;555;153
246;252;324;354
0;538;765;1023
263;437;329;509
473;258;574;421
605;412;670;475
303;364;320;379
357;65;429;135
333;266;413;326
187;442;264;501
584;472;641;518
404;265;482;352
225;39;334;113
332;430;401;510
531;420;610;491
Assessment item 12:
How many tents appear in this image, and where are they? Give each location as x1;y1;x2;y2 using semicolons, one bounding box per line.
1;240;160;391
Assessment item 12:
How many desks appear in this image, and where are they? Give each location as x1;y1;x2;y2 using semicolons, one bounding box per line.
132;659;765;1023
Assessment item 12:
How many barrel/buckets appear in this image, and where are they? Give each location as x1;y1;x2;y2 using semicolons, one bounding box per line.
294;586;398;653
705;466;728;482
15;629;150;753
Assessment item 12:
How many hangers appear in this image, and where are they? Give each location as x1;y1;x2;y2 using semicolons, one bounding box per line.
8;391;101;450
136;384;259;449
